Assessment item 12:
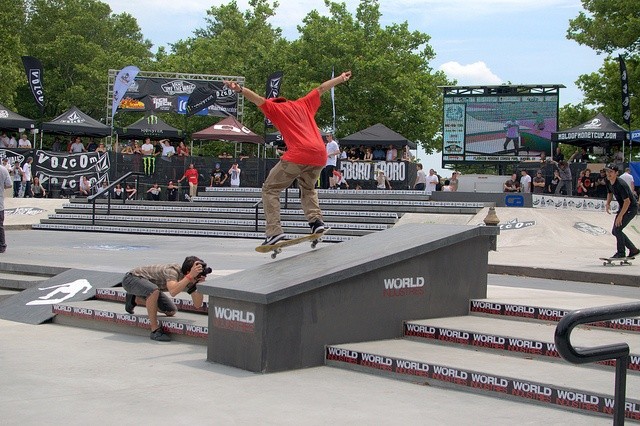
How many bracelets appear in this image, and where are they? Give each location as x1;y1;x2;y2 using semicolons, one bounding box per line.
235;85;243;93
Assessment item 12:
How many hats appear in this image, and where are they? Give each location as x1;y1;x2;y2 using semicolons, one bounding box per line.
604;163;619;172
268;97;286;102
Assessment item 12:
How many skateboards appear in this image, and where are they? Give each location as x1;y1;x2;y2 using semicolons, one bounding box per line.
184;193;191;201
598;255;636;267
255;225;331;259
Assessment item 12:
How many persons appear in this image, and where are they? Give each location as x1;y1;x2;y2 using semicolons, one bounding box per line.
553;148;564;161
605;163;640;259
69;137;88;154
23;157;35;198
375;171;392;190
533;169;546;194
503;173;520;192
8;133;17;148
613;144;624;163
18;133;31;148
457;171;462;175
618;168;635;195
449;172;459;192
585;169;592;182
185;275;193;282
335;168;350;189
152;140;162;155
413;163;426;190
373;144;386;160
573;147;589;161
114;184;124;199
61;140;69;150
600;146;612;156
400;144;412;162
363;148;373;161
101;183;111;198
532;110;545;130
94;142;106;152
441;180;453;192
125;183;137;201
433;171;443;191
67;137;76;151
548;169;563;194
175;141;188;157
51;137;61;151
217;151;233;158
209;162;228;187
0;156;13;252
596;169;607;198
141;138;153;155
113;139;141;154
86;136;99;152
177;163;199;198
426;169;439;191
583;177;592;197
30;176;46;198
338;146;348;159
577;171;586;196
147;182;162;201
555;160;573;196
122;256;212;342
347;144;360;163
519;169;532;193
80;176;92;196
237;153;249;160
358;144;365;160
228;161;242;187
224;70;353;248
0;132;10;148
504;113;520;155
386;144;398;162
276;148;283;157
11;161;23;198
1;158;12;173
321;132;341;189
159;138;175;156
165;180;178;201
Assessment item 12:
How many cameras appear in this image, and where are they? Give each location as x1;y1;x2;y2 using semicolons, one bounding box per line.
195;262;212;278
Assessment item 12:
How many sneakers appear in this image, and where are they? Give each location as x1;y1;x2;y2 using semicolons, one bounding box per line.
609;253;626;259
261;233;286;245
150;328;171;341
126;293;134;314
308;220;325;235
627;248;640;257
